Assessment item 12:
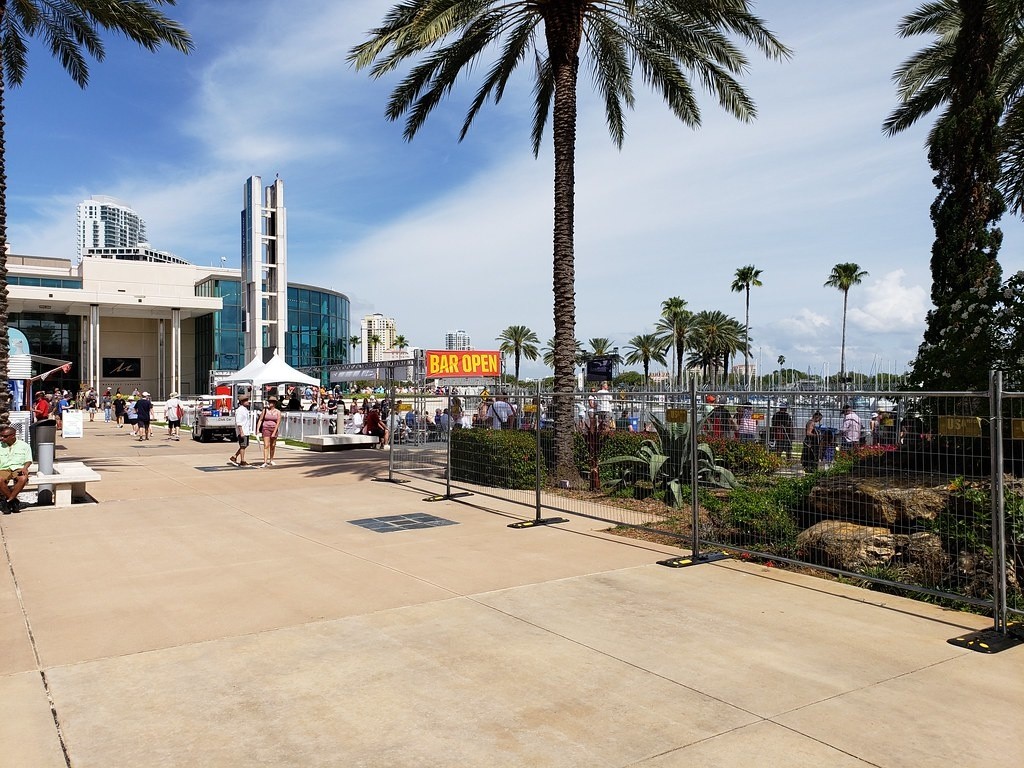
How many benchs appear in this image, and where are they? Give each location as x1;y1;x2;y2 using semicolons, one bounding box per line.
302;434;379;452
8;461;102;507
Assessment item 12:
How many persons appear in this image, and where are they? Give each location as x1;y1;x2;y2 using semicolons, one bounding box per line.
124;391;154;441
256;397;281;468
289;380;928;476
52;387;139;428
163;392;184;441
30;391;52;420
0;427;32;514
229;395;252;468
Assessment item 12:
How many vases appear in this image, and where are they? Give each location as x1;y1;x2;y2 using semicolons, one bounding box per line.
110;404;127;421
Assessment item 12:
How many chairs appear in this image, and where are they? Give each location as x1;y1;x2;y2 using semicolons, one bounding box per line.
435;416;448;441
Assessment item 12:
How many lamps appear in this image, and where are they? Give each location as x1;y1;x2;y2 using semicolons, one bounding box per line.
219;257;226;268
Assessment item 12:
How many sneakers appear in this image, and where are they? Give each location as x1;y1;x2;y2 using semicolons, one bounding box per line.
2;498;20;514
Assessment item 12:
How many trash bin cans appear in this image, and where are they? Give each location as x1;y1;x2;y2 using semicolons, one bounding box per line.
627;417;640;431
29;418;57;462
815;426;838;461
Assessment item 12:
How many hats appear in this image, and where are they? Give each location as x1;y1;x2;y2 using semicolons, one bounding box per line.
706;395;715;402
107;386;112;390
239;395;248;400
841;405;849;413
116;394;121;397
141;392;150;397
169;393;175;398
127;396;134;400
266;396;278;402
871;412;878;418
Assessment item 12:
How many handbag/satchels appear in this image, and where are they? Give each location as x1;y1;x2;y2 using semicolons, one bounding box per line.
177;404;184;421
501;421;508;430
259;421;264;432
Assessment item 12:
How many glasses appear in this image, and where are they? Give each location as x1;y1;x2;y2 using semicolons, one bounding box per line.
0;434;13;438
359;408;364;410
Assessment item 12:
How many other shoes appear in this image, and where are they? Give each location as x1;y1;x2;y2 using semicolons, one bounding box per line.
139;436;143;441
168;433;180;440
269;460;277;466
229;456;239;467
239;461;251;467
90;418;93;421
105;420;111;422
145;436;149;440
115;424;123;428
260;462;268;468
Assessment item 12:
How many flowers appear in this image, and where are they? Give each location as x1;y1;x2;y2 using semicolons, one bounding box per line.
111;395;129;405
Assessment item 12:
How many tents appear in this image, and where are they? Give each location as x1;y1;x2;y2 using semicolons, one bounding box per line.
217;348;320;411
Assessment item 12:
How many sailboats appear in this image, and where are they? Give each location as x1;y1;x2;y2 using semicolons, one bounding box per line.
653;354;913;413
